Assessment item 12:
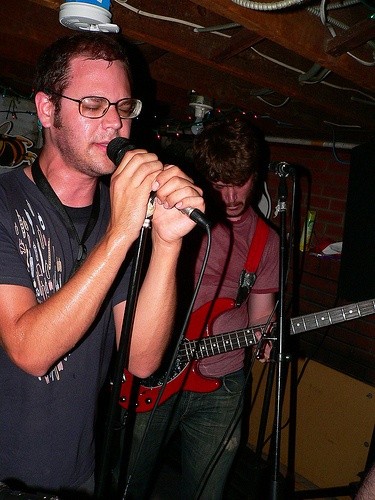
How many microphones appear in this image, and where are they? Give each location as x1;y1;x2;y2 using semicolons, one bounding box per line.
106;137;210;231
268;160;303;177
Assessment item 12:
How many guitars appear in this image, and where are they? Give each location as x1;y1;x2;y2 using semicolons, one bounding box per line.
109;297;374;414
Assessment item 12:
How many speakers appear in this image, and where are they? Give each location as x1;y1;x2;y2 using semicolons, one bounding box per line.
339;143;374;301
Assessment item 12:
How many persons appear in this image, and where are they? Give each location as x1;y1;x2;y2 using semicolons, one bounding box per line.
1;32;208;500
116;108;281;500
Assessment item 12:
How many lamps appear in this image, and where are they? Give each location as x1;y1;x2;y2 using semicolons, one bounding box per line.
188;95;213;135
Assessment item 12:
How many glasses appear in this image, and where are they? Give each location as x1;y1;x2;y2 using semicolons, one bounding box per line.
50;87;143;123
